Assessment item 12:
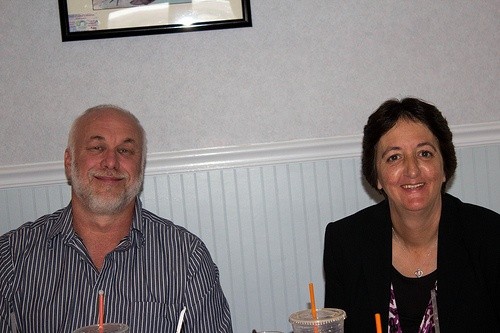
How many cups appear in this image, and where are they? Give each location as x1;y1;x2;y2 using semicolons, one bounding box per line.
74;323;129;333
289;308;346;333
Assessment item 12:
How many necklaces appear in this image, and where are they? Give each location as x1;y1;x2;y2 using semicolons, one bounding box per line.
392;226;437;279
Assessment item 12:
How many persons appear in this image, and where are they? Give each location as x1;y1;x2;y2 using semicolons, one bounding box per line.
323;98;500;333
0;103;233;333
